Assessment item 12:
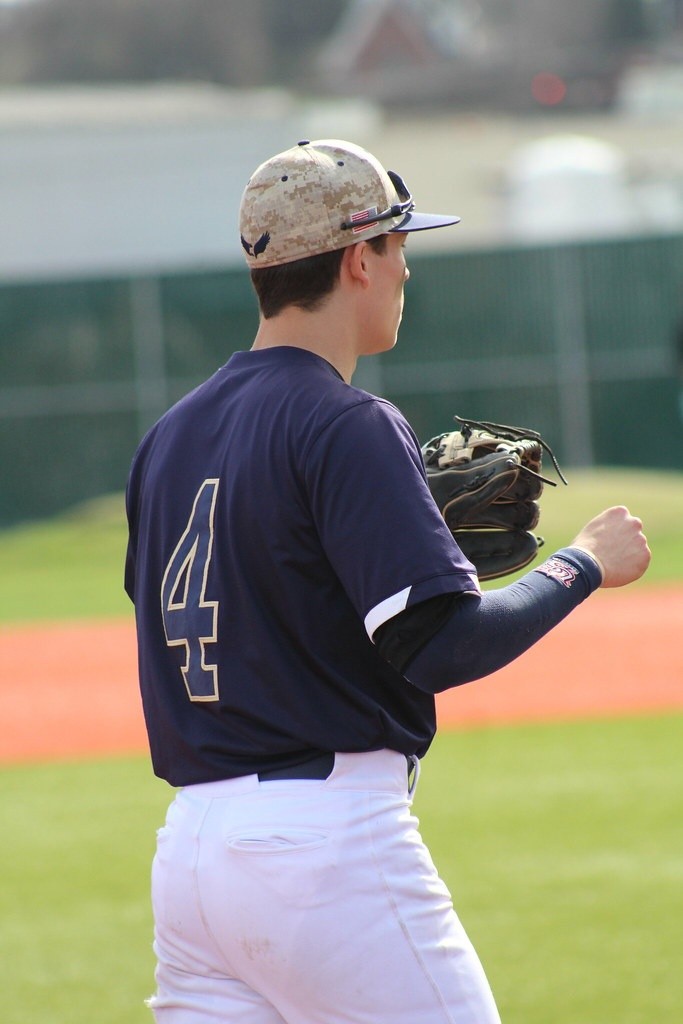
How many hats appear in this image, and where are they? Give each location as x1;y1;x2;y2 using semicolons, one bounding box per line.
239;139;462;270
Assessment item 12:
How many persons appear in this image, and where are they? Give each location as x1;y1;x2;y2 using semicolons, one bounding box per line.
126;140;651;1021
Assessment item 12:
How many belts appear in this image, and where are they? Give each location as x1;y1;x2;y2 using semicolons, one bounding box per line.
257;751;416;782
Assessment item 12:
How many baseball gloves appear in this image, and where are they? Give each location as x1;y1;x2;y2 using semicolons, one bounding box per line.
416;415;570;580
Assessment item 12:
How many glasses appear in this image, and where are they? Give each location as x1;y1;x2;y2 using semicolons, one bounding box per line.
341;171;416;230
